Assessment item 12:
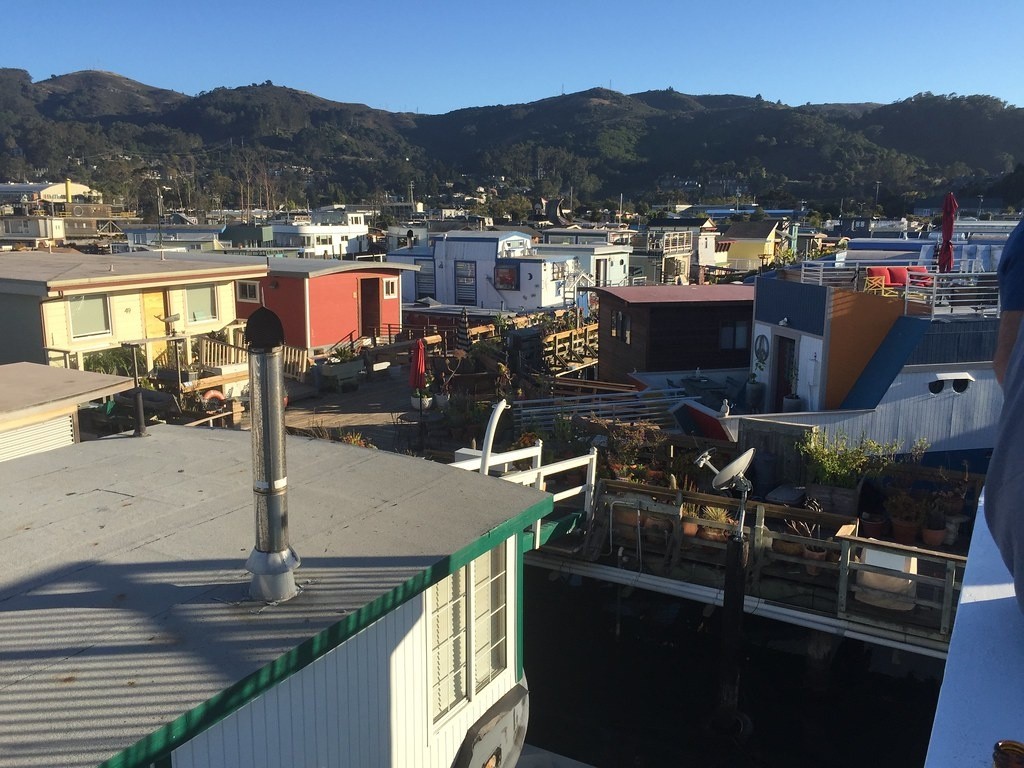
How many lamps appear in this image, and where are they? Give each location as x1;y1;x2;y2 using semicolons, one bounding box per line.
779;317;787;326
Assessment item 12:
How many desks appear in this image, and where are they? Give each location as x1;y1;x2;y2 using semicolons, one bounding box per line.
399;411;445;451
682;378;728;395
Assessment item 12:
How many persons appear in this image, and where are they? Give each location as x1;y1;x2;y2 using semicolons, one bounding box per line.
717;399;729;417
982;215;1024;611
696;368;700;379
632;368;637;375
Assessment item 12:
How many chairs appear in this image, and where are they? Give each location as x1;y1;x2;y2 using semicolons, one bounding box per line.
666;378;688;395
863;276;927;300
946;274;998;313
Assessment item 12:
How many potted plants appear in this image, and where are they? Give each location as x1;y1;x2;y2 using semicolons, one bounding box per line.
745;373;767;409
157;346;200;382
333;347;354;363
508;393;731;554
771;423;969;590
411;371;436;409
434;387;512;444
783;393;802;412
284;386;290;410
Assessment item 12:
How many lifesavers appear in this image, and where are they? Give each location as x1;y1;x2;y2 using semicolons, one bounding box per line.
201;390;226;416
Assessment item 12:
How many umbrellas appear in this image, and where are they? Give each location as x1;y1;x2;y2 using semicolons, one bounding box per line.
457;307;474;373
410;339;428;450
939;192;959;304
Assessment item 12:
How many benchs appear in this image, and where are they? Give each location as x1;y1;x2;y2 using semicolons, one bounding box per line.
866;266;945;305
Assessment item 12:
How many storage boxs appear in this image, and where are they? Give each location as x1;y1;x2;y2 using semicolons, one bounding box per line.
766;482;804;523
